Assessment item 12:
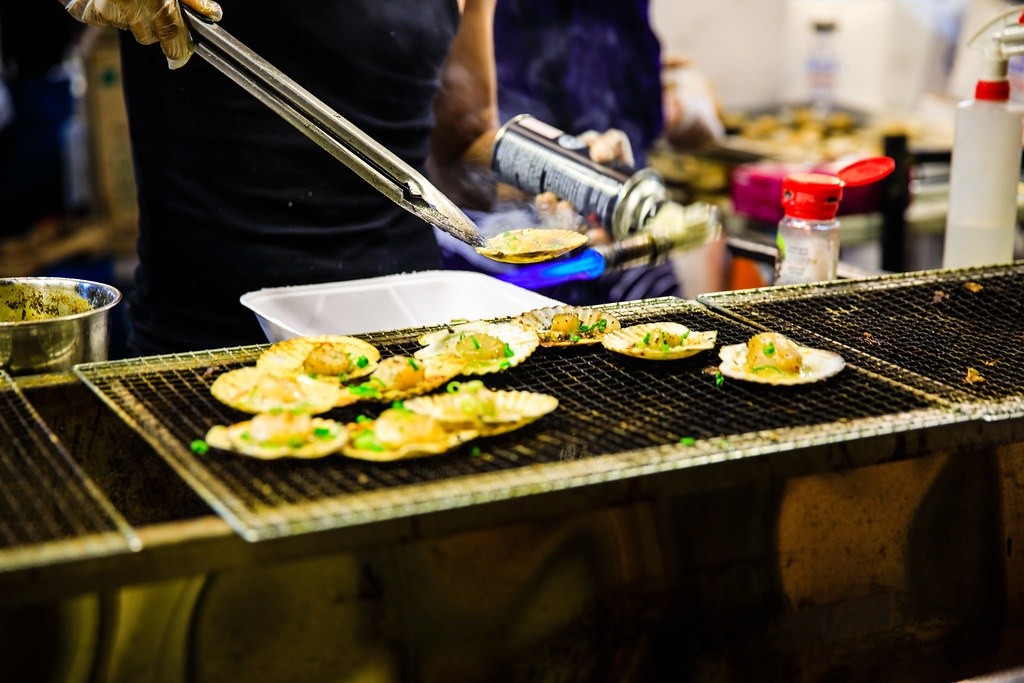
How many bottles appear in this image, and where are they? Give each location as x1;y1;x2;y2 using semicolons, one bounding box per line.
773;155;895;285
491;113;667;243
943;34;1024;270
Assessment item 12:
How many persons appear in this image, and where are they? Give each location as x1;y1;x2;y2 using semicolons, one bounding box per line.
426;0;723;310
0;0;90;222
60;0;635;369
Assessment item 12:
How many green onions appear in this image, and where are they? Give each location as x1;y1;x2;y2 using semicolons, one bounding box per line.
191;316;776;451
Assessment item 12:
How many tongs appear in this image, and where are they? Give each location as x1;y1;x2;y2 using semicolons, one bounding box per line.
179;2;488;251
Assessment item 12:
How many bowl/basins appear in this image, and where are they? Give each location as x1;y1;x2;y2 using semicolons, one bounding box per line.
0;277;124;377
